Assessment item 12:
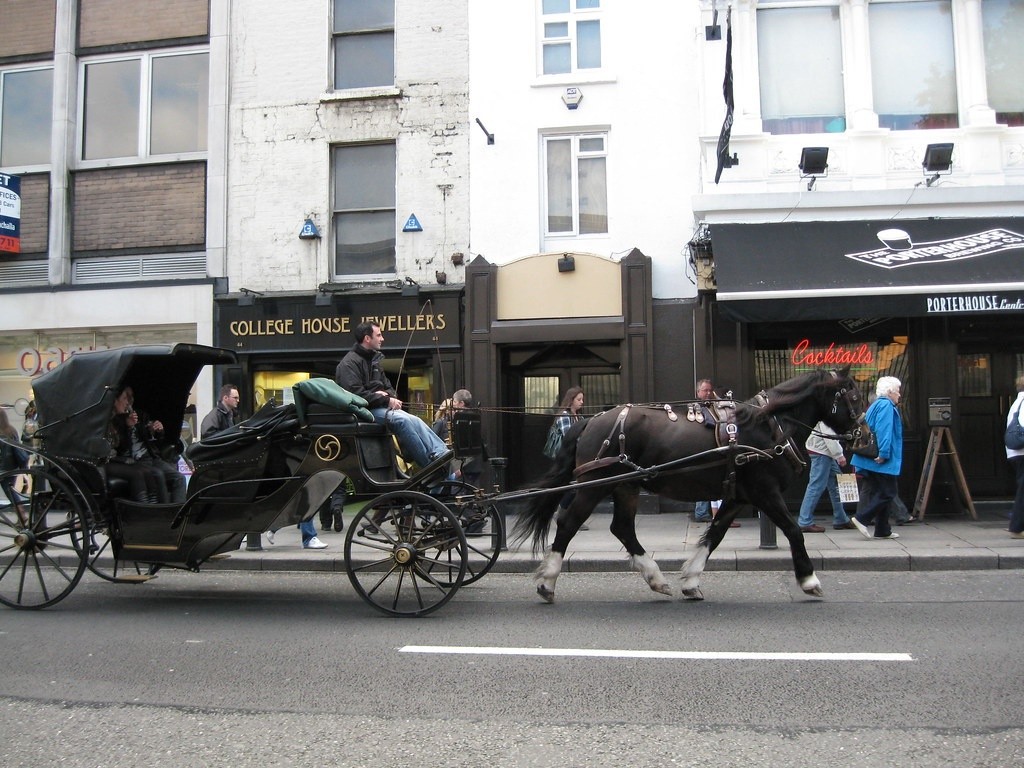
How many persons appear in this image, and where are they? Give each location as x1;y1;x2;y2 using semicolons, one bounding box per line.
266;518;328;548
695;379;741;527
1006;377;1024;537
320;478;346;532
201;385;247;542
336;321;449;467
432;390;482;537
543;388;589;530
852;376;917;538
798;421;856;531
360;509;417;534
106;386;186;504
182;421;197;446
0;401;41;525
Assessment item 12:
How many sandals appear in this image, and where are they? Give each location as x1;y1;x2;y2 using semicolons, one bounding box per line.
896;515;917;525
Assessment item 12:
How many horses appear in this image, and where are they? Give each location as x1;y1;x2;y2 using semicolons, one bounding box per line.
518;362;880;603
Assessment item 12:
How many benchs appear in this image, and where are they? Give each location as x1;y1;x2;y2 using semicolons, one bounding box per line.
95;463;132;499
304;402;387;437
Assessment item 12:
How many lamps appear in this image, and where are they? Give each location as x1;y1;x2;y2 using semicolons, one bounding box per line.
314;288;359;306
402;277;422;296
557;251;575;271
238;288;264;306
923;143;954;186
800;147;829;190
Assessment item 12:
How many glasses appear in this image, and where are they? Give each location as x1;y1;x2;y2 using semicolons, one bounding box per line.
228;396;239;399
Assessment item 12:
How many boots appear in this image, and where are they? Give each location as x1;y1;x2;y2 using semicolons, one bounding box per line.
553;504;569;525
579;524;588;530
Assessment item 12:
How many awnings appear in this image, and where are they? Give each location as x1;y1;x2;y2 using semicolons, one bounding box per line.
709;218;1024;322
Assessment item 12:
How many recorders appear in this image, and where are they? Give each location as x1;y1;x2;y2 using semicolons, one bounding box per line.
928;397;952;426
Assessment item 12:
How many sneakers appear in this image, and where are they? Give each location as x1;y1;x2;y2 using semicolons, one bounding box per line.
302;537;328;548
266;530;274;544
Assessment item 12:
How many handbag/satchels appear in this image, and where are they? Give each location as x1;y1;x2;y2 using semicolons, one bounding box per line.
15;447;29;468
1005;412;1024;450
837;473;859;503
543;427;567;460
853;432;878;458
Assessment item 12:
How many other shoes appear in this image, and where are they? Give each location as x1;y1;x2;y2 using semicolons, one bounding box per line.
731;522;740;527
1008;531;1024;539
800;524;826;532
697;517;713;522
874;532;899;539
333;508;343;532
361;521;378;534
834;522;856;529
852;517;871;538
321;523;332;531
